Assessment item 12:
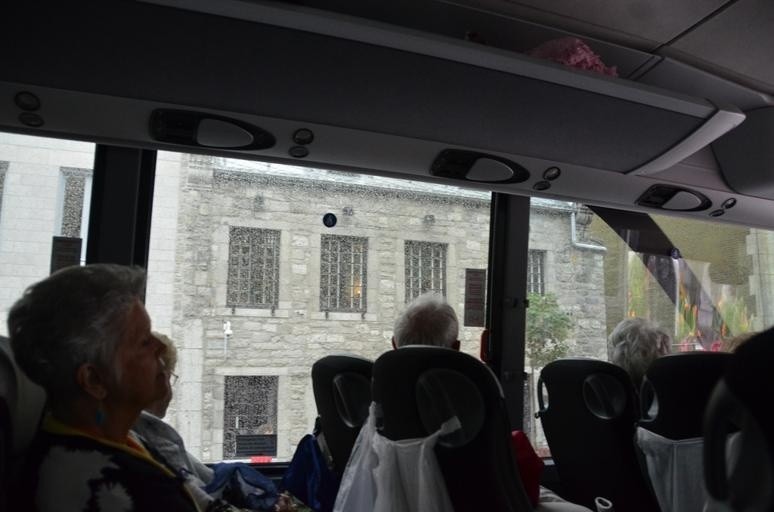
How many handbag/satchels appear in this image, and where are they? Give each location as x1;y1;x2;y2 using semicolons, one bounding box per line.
278;417;340;511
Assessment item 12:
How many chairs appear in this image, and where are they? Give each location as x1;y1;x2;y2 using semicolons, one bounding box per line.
3;338;51;512
535;357;639;512
695;336;774;511
307;353;374;484
372;345;535;512
639;350;732;512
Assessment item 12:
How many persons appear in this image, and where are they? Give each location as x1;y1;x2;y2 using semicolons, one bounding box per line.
391;295;463;353
720;332;762;353
132;334;300;512
606;317;673;421
6;260;252;511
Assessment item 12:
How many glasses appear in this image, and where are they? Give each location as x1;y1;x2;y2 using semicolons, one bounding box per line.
164;365;178;387
